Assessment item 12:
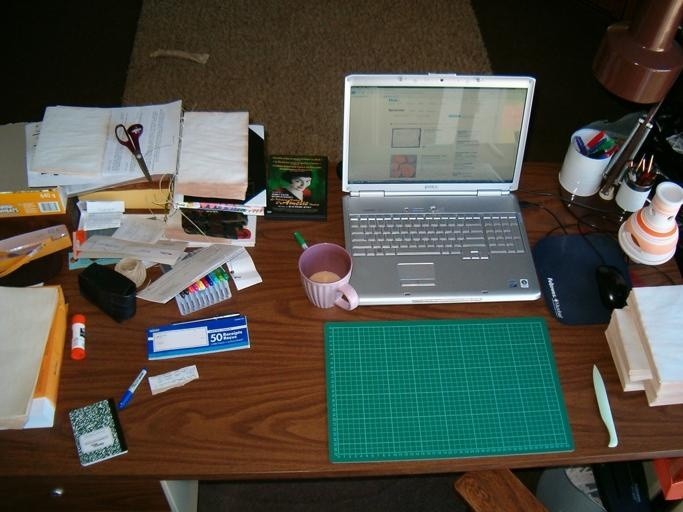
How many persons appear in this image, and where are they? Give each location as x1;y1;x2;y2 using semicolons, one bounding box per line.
268;169;320;210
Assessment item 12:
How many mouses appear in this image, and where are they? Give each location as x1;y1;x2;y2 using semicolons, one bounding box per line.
596;266;627;309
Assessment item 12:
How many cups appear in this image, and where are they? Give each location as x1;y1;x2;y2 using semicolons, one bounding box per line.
298;242;360;313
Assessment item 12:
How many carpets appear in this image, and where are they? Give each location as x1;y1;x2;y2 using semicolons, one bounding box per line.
121;0;497;163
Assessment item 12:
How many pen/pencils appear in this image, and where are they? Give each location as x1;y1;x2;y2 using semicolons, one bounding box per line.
628;153;656;186
161;249;229;298
294;232;308;250
118;368;148;409
576;132;619;159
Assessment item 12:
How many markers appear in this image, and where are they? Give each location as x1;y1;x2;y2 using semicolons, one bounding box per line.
72;314;86;360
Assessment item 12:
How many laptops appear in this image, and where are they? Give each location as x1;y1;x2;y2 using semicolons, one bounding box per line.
342;75;541;306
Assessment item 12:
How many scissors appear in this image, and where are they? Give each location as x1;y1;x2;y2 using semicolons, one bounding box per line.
115;124;151;184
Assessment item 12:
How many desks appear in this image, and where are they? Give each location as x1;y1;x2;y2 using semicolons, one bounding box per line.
0;148;683;511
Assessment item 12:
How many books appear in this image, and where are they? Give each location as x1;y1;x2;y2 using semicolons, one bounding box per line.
66;396;129;470
24;98;266;270
261;153;329;221
